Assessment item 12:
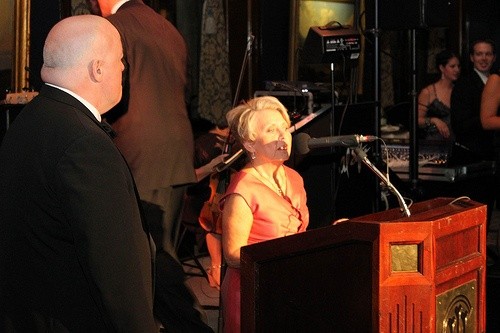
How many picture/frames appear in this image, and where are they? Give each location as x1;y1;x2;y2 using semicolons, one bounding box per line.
0;0;31;92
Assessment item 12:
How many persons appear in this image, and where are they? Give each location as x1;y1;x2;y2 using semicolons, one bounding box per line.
0;15;160;333
418;40;500;232
94;0;229;291
220;96;350;333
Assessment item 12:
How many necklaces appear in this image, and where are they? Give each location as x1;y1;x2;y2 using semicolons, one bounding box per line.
249;162;283;193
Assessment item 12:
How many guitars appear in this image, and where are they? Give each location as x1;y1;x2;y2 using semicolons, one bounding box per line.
198;100;254;234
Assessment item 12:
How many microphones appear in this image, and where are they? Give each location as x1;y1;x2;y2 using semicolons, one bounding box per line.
293;133;379;154
